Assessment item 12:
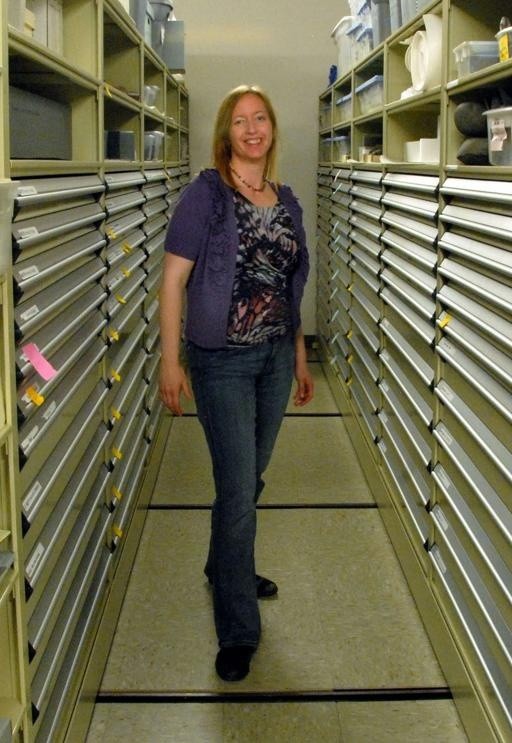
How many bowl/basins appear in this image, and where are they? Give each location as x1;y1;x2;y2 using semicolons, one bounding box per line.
229;164;266;192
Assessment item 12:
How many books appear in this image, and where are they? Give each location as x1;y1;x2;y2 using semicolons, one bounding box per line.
319;0;436;162
482;107;512;166
452;41;499;78
144;84;164;161
104;130;135;161
494;27;512;58
118;0;186;74
8;0;72;160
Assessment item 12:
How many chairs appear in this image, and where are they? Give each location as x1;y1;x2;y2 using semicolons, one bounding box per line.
255;573;278;596
215;644;256;681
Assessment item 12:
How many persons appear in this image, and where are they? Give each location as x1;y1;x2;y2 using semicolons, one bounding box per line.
157;83;313;681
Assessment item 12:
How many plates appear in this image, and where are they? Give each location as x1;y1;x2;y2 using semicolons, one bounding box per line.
315;0;512;743
0;0;192;743
